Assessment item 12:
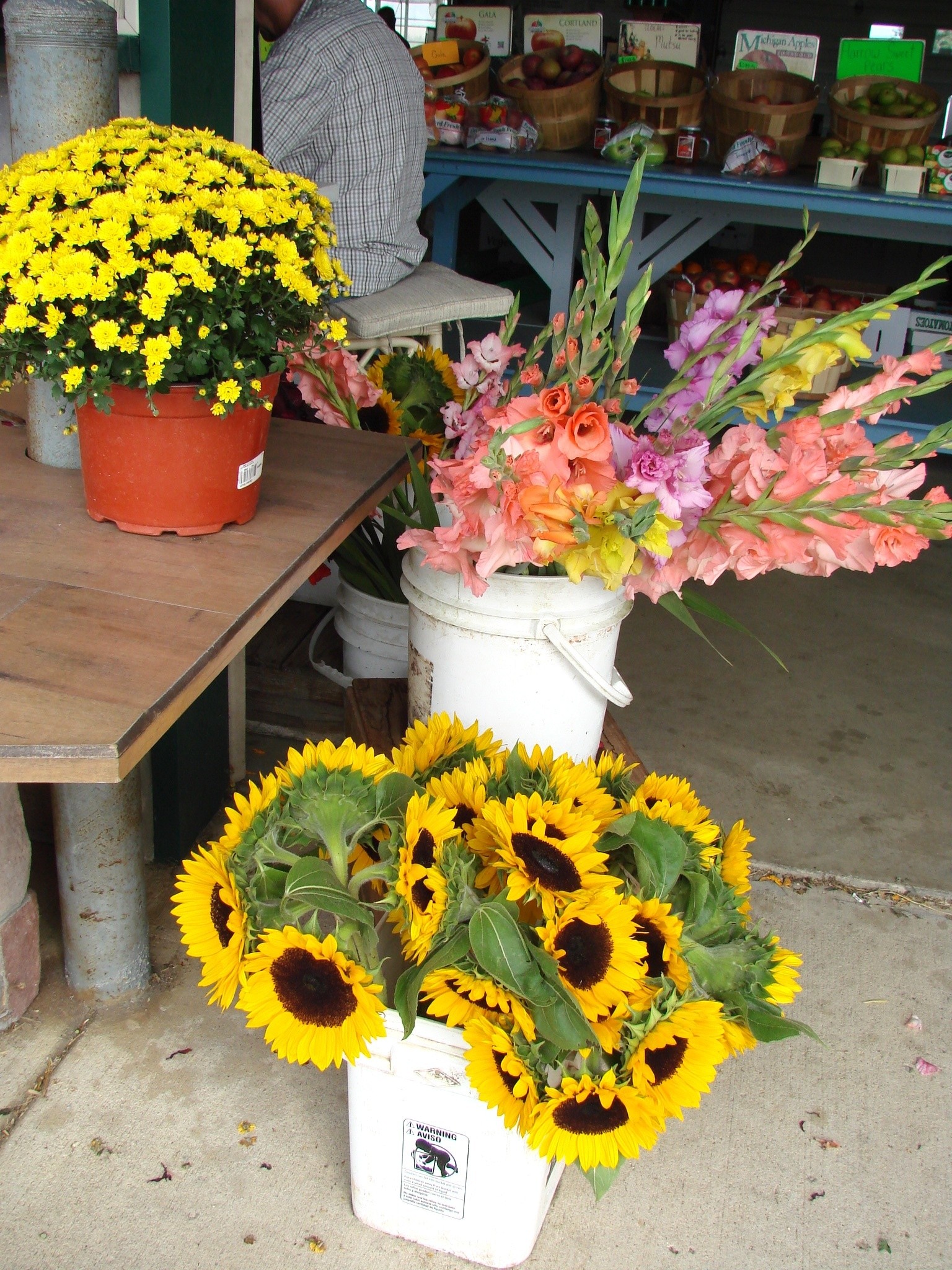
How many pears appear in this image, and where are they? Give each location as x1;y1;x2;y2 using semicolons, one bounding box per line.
879;143;925;166
842;81;937;119
820;137;870;163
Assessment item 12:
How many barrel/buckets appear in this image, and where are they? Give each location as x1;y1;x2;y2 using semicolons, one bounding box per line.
602;59;710;157
408;38;491;104
308;567;409;688
750;304;853;401
398;500;635;764
345;1009;565;1269
664;286;767;349
827;75;944;187
497;49;606;152
706;68;822;169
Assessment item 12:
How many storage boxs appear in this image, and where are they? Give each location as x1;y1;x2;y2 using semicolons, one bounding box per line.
876;160;927;195
846;293;952;365
815;157;869;186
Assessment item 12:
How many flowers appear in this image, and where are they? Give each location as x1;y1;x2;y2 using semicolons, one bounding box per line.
0;117;952;672
168;712;811;1196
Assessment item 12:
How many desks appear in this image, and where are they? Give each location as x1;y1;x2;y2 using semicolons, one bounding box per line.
1;364;415;994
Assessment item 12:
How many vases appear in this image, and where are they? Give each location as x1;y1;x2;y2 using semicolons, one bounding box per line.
72;365;286;539
349;1010;565;1267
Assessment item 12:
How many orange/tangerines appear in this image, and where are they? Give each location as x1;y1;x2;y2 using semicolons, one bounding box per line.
671;252;772;274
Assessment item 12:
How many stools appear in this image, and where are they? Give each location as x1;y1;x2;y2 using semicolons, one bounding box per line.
302;260;514;365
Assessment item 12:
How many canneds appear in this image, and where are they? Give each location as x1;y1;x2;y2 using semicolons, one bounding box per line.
673;125;700;167
590;118;616;154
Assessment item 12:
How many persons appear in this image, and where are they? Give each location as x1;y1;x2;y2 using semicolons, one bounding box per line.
254;0;429;302
378;7;411;50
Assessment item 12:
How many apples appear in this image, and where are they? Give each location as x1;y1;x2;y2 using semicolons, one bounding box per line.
604;120;667;165
508;44;594;90
413;47;480;80
531;29;566;51
673;269;877;313
722;132;789;177
752;94;794;105
735;49;789;72
423;97;534;153
444;15;477;39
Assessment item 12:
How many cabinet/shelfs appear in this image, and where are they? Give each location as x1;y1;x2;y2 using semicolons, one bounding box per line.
414;143;952;458
